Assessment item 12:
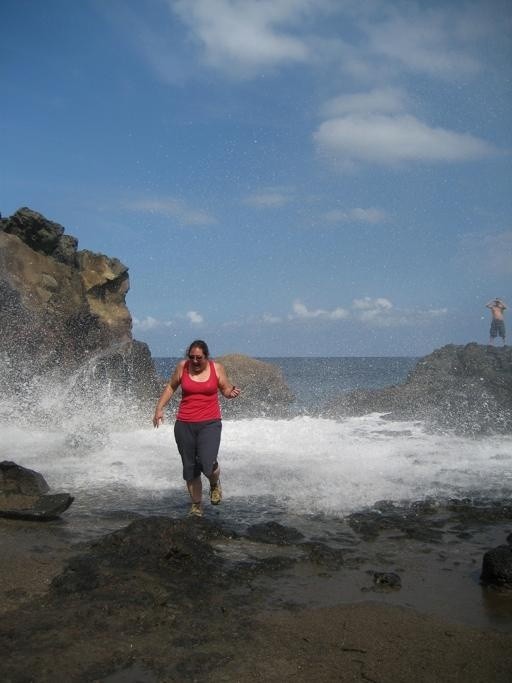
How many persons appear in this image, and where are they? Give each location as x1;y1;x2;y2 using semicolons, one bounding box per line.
484;297;509;346
152;339;242;518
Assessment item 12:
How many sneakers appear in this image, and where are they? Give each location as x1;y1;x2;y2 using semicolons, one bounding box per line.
190;500;203;517
210;479;220;504
